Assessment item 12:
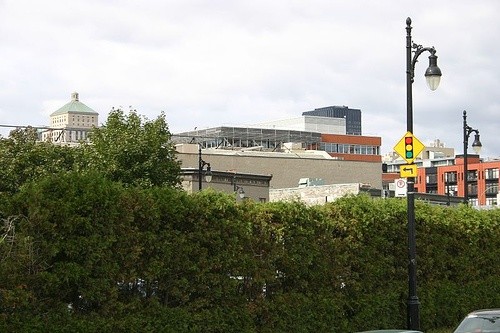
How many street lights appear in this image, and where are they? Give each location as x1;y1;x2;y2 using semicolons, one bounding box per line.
234;177;245;204
198;148;212;192
405;16;443;333
462;110;482;204
